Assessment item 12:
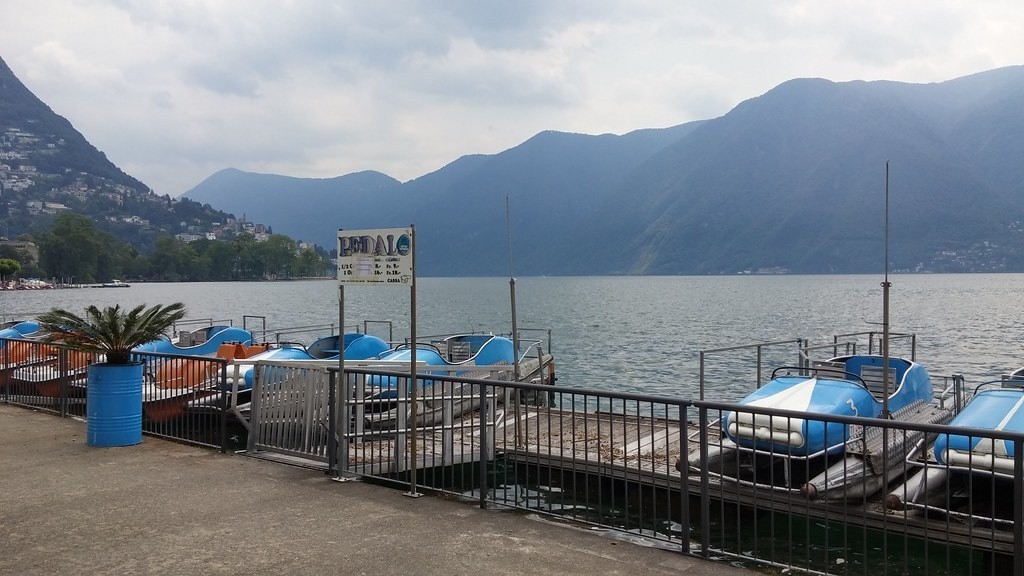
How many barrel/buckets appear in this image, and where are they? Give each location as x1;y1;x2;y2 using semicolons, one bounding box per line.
85;362;144;447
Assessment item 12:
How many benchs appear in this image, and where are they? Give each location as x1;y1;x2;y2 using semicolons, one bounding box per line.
320;333;364;353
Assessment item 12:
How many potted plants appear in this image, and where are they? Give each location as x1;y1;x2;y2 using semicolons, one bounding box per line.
34;303;189;447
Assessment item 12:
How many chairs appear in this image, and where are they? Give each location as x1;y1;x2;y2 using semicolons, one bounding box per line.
811;361;847;381
195;331;207;346
1001;375;1024;388
860;365;897;401
431;340;449;362
451;342;471;363
178;331;191;347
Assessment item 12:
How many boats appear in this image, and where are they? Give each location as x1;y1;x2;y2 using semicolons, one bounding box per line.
677;331;970;504
0;314;553;442
886;366;1024;524
